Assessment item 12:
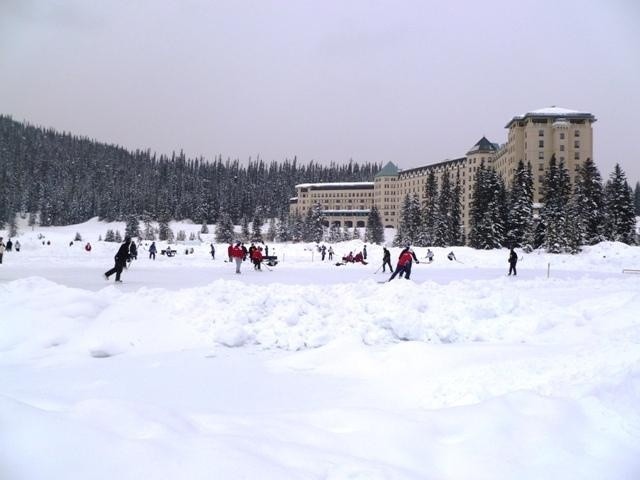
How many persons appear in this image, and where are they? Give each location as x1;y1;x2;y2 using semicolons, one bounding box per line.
322;249;327;261
6;239;12;252
398;246;420;281
363;245;368;259
0;236;6;265
327;246;334;260
347;251;354;262
148;242;157;261
426;248;434;260
210;244;215;260
234;242;244;274
241;242;264;272
104;235;136;284
507;247;518;276
228;243;233;263
356;251;362;262
388;249;416;282
447;250;456;261
130;241;137;260
85;242;91;251
382;248;394;273
15;240;21;252
